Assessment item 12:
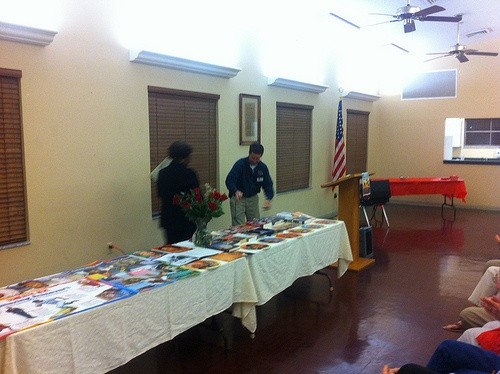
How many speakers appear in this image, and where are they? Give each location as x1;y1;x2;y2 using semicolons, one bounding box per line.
359;226;373;258
359;179;390;206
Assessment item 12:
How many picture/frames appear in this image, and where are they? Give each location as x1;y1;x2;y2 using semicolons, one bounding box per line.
238;93;262;146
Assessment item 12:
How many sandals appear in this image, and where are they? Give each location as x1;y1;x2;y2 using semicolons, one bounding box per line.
442;320;465;332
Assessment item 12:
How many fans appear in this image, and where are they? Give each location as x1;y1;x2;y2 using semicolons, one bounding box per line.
367;0;463;33
422;19;498;64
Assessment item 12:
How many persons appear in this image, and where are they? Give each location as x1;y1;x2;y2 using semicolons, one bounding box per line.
380;234;500;374
157;143;206;245
225;143;275;225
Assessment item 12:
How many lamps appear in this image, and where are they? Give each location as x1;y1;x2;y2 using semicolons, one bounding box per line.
267;75;328;94
129;49;241;78
0;20;57;47
341;90;381;101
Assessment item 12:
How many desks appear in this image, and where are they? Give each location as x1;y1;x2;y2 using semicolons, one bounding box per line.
371;177;467;219
0;214;356;374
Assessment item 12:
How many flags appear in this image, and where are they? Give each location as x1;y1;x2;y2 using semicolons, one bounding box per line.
331;100;346;198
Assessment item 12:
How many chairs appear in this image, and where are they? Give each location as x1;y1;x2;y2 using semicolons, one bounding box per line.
361;180;392;229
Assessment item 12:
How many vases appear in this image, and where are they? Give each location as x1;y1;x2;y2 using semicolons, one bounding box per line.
192;221;212;247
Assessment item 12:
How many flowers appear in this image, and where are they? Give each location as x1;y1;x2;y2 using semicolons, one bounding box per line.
175;185;227;220
